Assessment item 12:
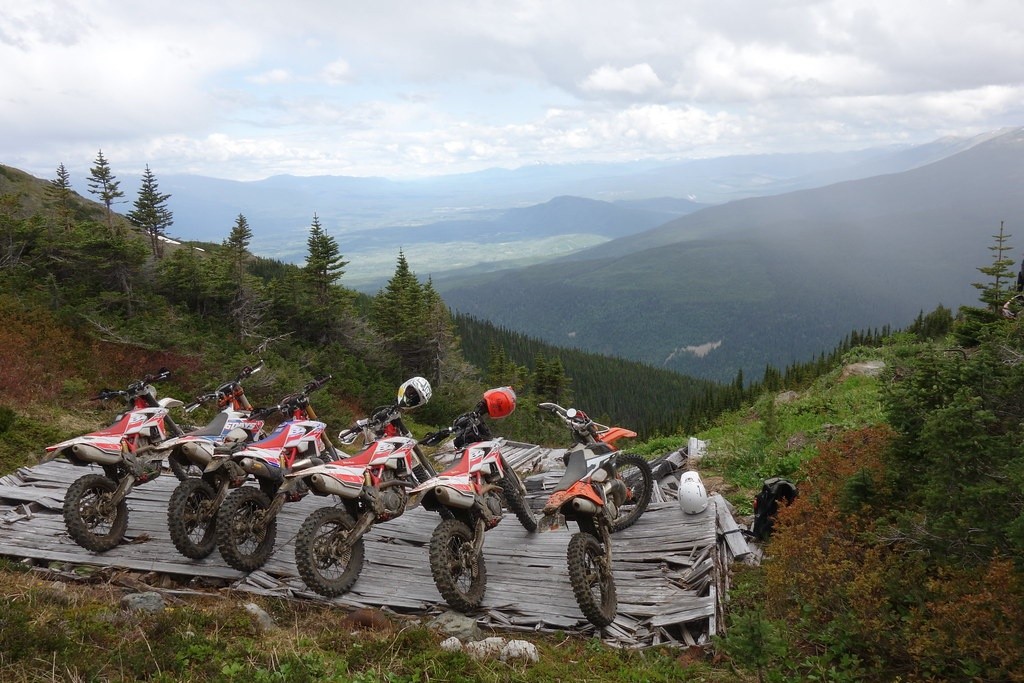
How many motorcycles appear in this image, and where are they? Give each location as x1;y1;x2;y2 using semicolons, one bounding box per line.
536;401;654;629
40;366;202;554
155;359;303;561
203;372;341;573
417;384;538;614
294;376;457;598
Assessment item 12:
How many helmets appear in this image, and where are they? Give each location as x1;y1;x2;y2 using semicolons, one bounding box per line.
677;471;708;515
397;377;432;411
484;386;517;420
